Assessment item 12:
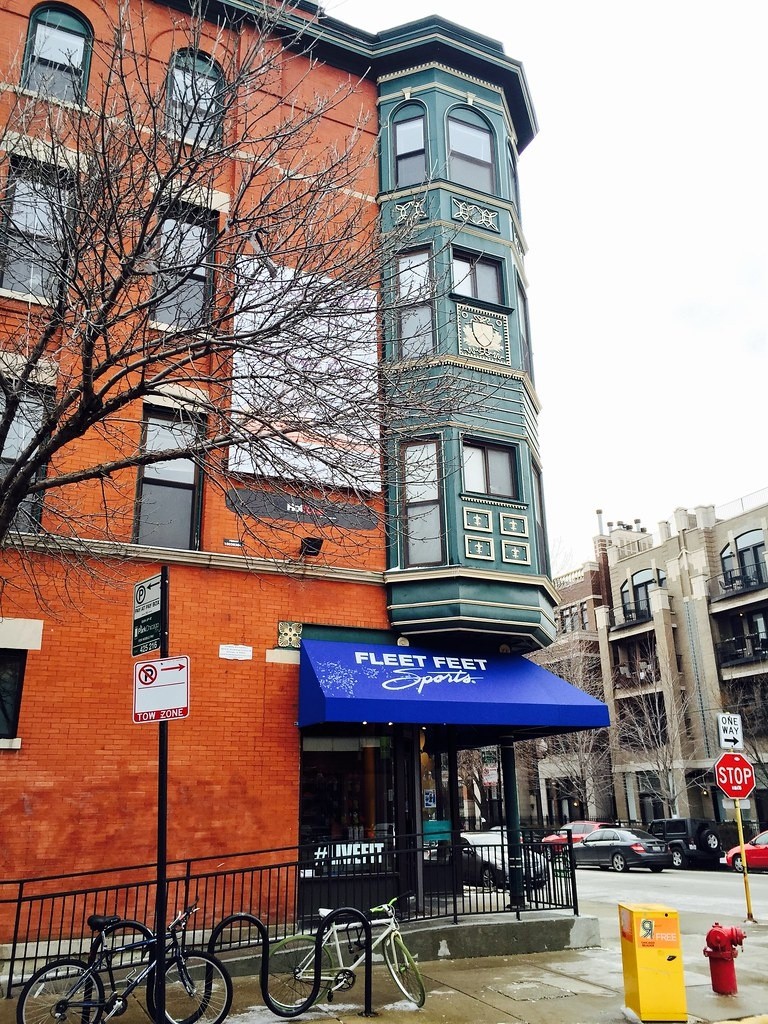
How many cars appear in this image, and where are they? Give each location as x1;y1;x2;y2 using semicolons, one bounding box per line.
489;826;543;853
540;819;619;857
561;824;670;874
726;829;768;870
442;830;551;891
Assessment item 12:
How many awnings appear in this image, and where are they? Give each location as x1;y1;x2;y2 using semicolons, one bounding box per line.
296;636;613;738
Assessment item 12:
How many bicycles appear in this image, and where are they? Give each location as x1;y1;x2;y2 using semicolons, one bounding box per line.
260;888;426;1017
14;898;233;1024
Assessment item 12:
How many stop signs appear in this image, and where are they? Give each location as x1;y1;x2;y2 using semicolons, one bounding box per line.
715;753;755;799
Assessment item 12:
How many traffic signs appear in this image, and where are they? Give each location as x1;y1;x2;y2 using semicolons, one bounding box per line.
718;712;744;749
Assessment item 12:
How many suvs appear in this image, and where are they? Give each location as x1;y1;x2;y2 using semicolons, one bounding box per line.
648;819;719;866
